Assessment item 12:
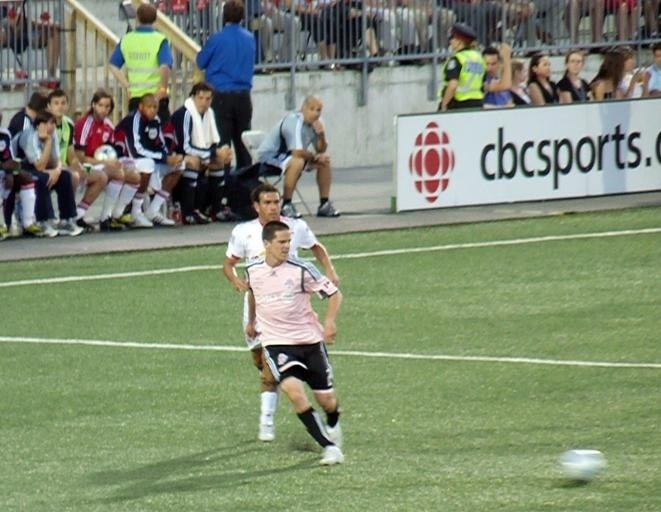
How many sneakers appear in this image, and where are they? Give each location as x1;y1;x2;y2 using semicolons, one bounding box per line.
317;201;340;217
258;422;343;466
281;202;303;218
0;205;242;241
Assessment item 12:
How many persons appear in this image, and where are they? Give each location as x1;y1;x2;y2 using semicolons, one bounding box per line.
223;185;339;442
0;0;341;240
246;220;345;465
339;1;660;113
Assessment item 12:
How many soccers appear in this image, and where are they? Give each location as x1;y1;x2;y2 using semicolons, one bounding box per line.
95;144;117;162
564;449;603;482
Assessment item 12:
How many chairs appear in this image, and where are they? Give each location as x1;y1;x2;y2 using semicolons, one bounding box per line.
118;1;313;72
240;130;311;216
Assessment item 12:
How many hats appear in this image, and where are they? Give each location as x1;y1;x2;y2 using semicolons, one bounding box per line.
451;22;478;43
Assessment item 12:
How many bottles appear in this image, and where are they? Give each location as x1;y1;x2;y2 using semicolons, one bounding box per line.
173;205;180;223
223;204;229;219
391;196;396;213
